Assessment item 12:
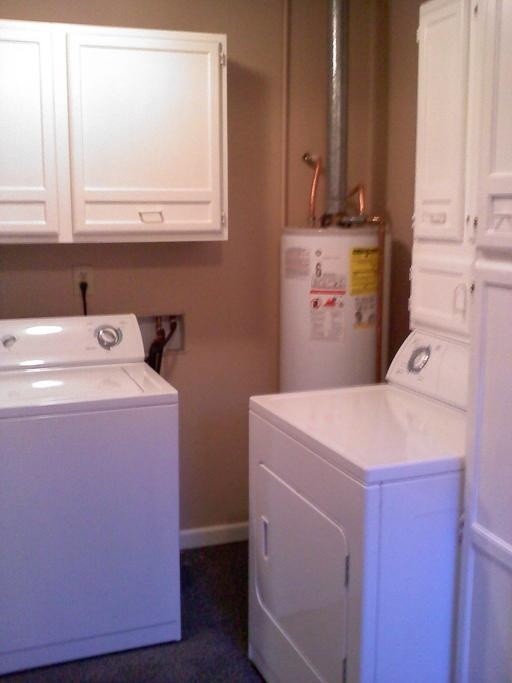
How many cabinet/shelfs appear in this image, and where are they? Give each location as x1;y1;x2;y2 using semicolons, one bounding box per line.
411;0;511;250
64;26;228;242
408;246;474;342
458;261;510;682
0;19;64;244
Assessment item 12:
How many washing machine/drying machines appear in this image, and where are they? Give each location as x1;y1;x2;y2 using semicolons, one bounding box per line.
0;313;184;676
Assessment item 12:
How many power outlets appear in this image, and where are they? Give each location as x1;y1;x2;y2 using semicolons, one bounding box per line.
72;264;92;297
